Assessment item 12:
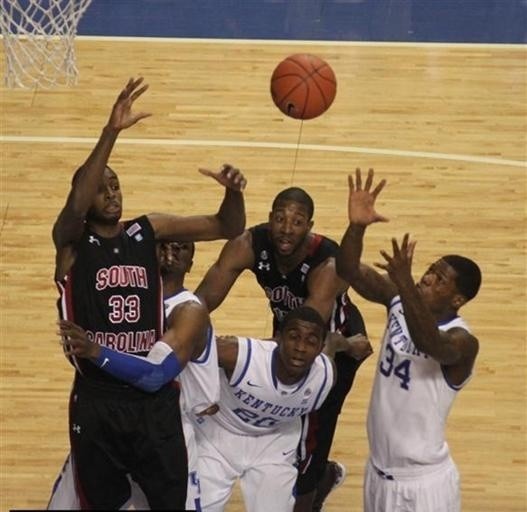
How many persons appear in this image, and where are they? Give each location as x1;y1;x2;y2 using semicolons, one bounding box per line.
198;304;372;512
192;187;373;512
50;74;247;512
334;167;484;511
46;240;221;511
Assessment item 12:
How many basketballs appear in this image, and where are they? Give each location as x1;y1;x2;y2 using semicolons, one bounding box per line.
267;51;339;121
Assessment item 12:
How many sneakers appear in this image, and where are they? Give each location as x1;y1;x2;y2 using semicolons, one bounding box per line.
313;461;345;512
